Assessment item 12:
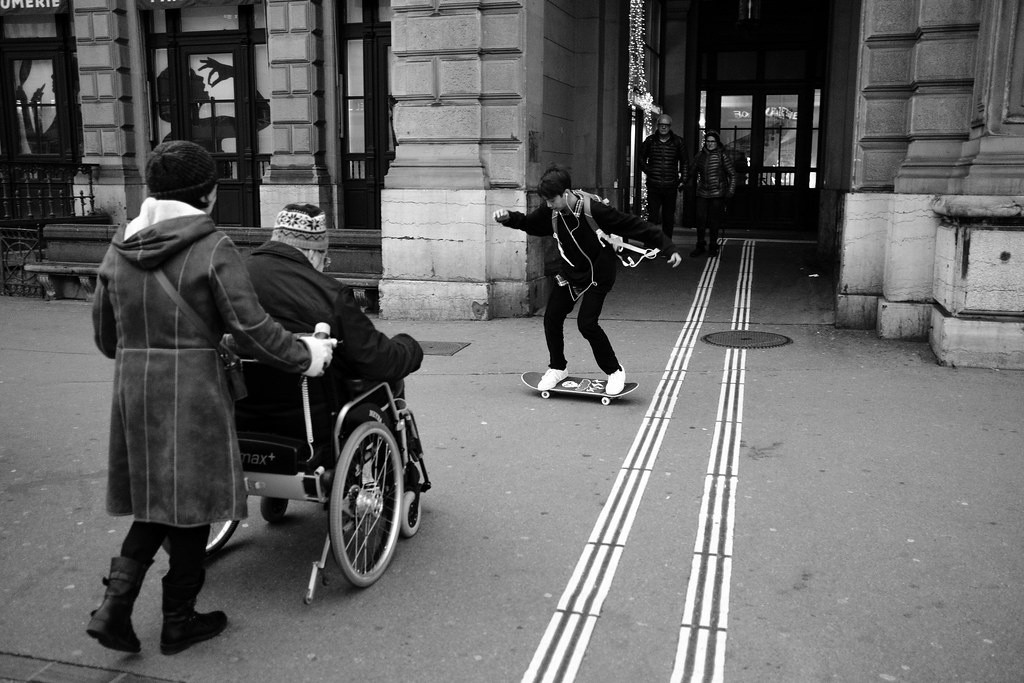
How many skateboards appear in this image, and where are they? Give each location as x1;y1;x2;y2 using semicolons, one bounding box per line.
521;370;640;406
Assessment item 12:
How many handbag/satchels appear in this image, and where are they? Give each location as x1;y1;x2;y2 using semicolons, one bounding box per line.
220;353;247;401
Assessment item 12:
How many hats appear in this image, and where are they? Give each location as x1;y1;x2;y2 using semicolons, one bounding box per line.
145;141;216;202
271;204;329;251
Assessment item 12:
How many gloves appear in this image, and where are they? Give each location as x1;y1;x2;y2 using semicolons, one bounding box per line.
676;179;688;193
726;191;734;198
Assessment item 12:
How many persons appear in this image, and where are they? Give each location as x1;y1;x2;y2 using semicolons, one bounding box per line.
223;203;423;524
86;140;337;655
689;130;736;257
636;113;689;259
495;168;683;395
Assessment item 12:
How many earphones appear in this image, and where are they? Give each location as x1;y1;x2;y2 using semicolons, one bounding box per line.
565;193;568;195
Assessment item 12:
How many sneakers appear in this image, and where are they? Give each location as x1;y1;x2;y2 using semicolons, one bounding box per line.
606;363;626;395
538;366;568;390
660;250;665;257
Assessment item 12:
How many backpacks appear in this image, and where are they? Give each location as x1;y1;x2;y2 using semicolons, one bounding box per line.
552;190;656;267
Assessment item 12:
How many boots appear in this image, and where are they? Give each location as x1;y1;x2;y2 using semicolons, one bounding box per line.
86;557;155;653
160;574;227;655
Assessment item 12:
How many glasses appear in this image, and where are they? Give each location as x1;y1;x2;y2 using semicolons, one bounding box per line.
658;123;671;127
319;254;331;267
706;140;716;143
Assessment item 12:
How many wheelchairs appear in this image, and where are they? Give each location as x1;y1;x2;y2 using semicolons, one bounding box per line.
152;325;431;608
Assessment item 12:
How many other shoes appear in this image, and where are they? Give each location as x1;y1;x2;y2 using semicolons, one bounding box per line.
708;243;719;256
690;241;707;257
